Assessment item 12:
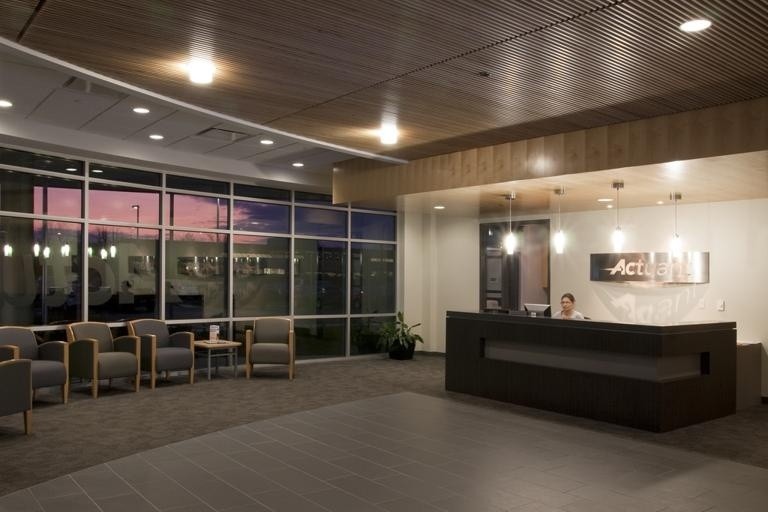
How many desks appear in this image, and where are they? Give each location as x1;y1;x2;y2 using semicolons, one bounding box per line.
191;334;243;384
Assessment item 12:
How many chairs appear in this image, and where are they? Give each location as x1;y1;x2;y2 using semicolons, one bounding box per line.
126;316;198;394
64;321;144;403
1;323;73;405
243;314;297;382
0;352;35;441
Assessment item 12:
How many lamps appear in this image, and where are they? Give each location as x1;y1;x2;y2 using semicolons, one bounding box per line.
0;229;122;261
500;189;522;258
606;178;627;255
666;191;690;260
548;187;573;255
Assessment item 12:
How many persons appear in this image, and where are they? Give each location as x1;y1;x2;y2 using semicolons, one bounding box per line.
67;280;86;294
550;291;583;320
118;279;136;295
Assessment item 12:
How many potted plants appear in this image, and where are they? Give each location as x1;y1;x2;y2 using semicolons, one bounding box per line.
376;311;425;361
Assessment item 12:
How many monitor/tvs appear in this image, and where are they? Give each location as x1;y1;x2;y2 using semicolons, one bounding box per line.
524;304;551;317
